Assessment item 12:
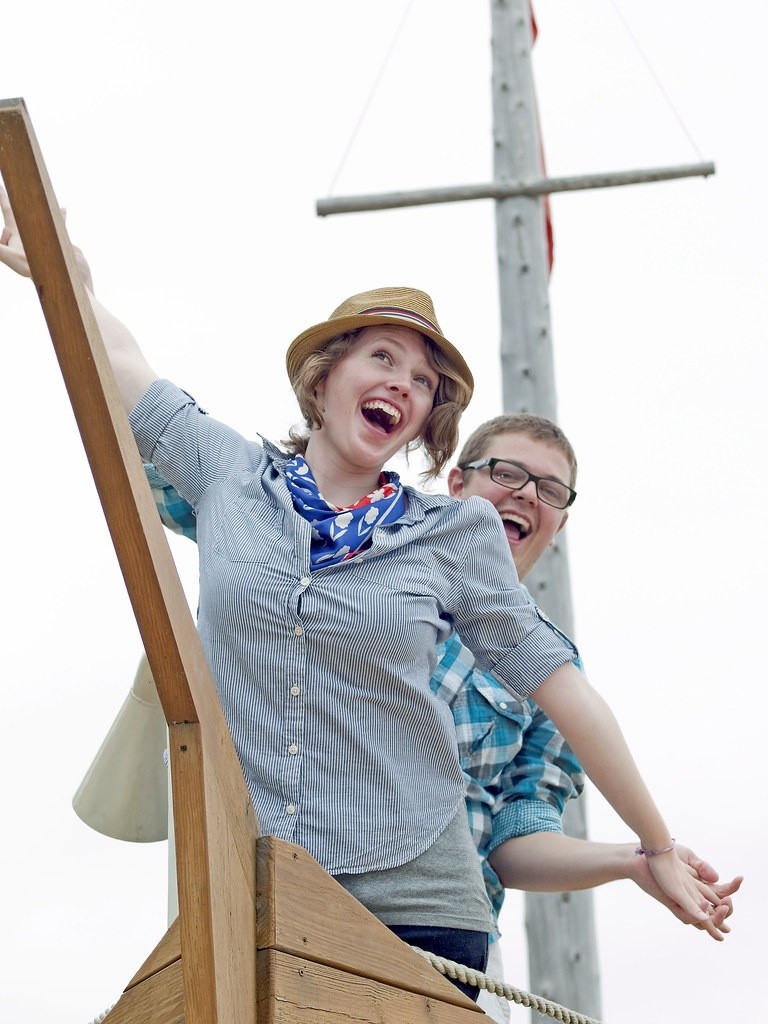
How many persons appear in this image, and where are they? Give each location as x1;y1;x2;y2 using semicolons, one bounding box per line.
0;193;745;1004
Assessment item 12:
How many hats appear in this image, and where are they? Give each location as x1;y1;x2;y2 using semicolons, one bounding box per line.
287;286;474;410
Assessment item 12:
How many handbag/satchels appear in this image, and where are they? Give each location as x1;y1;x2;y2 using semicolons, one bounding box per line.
70;651;169;844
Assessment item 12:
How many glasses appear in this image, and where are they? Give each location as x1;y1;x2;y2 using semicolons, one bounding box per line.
461;457;578;509
